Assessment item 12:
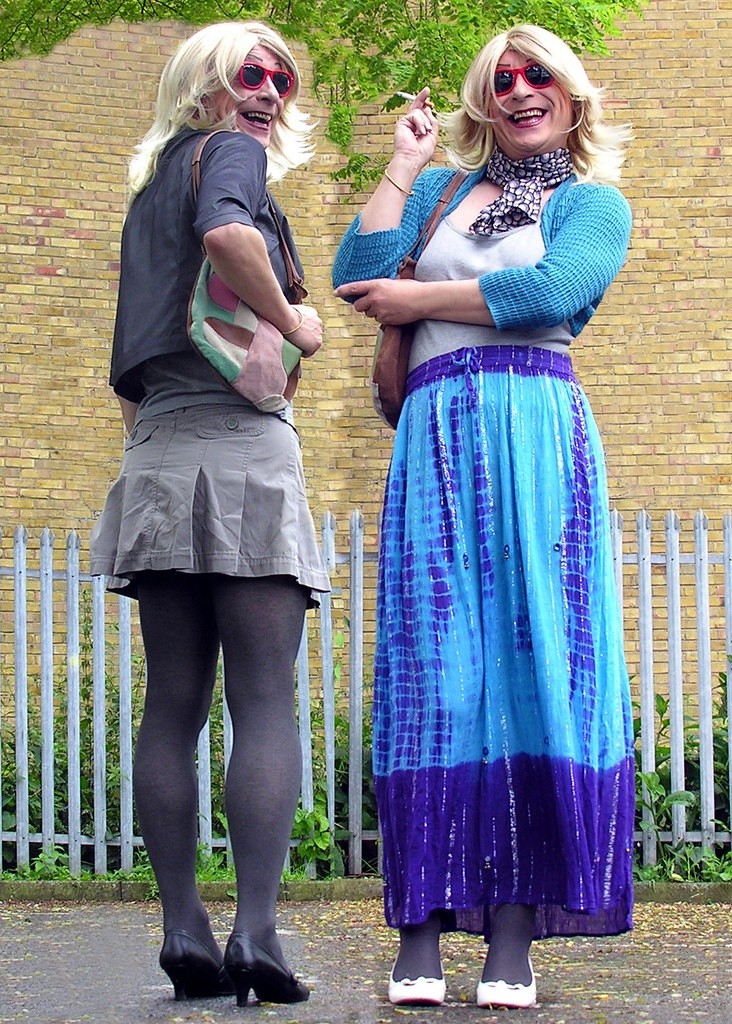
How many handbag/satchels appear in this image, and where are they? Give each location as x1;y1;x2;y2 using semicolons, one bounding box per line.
370;167;470;429
187;127;306;413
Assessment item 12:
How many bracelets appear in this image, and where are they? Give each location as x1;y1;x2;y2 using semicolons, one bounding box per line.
383;169;412;196
281;303;303;335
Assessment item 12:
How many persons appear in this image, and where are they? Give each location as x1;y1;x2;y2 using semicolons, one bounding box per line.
104;18;311;1007
328;23;636;1009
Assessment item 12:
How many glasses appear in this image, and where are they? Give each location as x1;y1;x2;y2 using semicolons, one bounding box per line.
485;63;554;95
238;63;295;98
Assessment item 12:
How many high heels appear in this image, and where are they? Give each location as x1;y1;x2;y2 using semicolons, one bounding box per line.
222;933;309;1007
159;929;238;1000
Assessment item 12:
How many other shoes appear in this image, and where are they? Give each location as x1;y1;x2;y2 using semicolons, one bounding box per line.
476;956;537;1009
387;951;446;1007
